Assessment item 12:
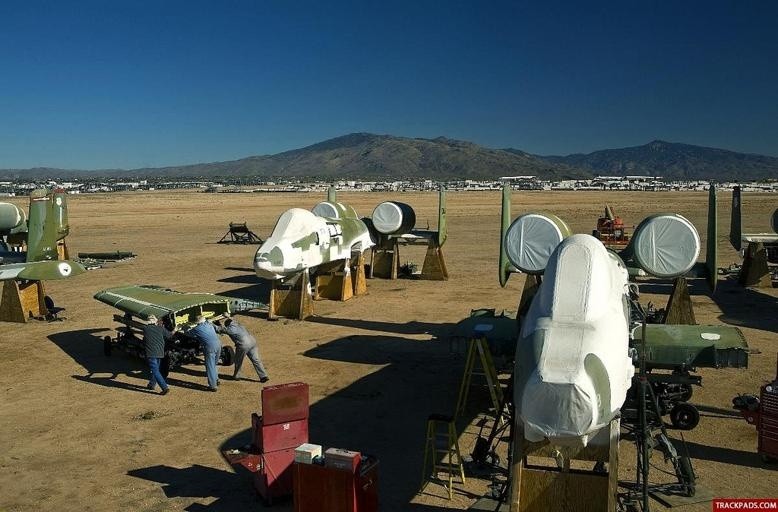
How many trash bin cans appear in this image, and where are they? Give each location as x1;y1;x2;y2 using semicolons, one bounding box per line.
419;413;466;501
453;334;509;431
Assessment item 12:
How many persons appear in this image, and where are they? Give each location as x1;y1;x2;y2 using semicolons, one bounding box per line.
210;311;270;383
142;314;178;396
185;315;223;392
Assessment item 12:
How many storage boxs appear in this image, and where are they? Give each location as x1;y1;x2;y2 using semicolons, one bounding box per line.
326;447;362;472
293;443;322;464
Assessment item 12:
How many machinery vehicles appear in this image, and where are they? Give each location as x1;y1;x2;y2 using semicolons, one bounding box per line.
592;205;636;254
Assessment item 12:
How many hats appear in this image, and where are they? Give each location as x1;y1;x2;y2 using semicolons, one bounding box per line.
194;315;206;324
147;315;158;325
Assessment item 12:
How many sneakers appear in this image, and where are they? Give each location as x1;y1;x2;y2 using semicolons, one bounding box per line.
207;386;218;392
147;384;154;390
159;389;170;395
261;377;269;383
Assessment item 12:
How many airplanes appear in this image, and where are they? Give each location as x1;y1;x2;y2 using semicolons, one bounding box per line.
728;185;778;257
460;177;720;511
0;185;91;281
244;184;450;284
